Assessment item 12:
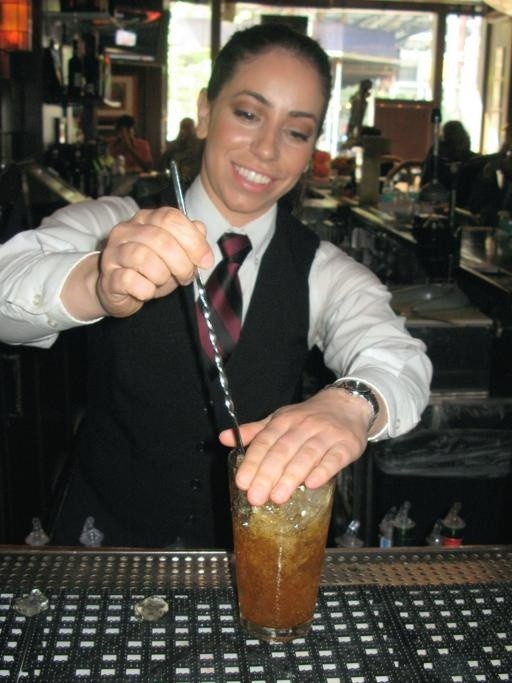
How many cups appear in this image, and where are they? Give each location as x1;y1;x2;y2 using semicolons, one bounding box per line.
226;444;338;640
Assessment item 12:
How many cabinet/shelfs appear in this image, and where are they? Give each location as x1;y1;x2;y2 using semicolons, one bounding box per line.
32;1;165;201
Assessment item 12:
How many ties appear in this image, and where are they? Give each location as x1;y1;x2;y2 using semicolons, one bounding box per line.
191;232;252;367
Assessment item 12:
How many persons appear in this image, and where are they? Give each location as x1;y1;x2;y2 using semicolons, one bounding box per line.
0;23;437;549
415;118;477;190
99;112;155;173
163;116;202;172
342;74;375;136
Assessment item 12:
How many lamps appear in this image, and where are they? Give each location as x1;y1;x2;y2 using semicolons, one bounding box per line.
0;0;30;53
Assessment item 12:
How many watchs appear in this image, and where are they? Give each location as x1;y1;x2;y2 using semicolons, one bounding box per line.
326;379;378;433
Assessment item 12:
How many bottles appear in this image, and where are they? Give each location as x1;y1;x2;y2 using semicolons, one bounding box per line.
338;504;468;548
67;28;100;99
54;137;114;198
